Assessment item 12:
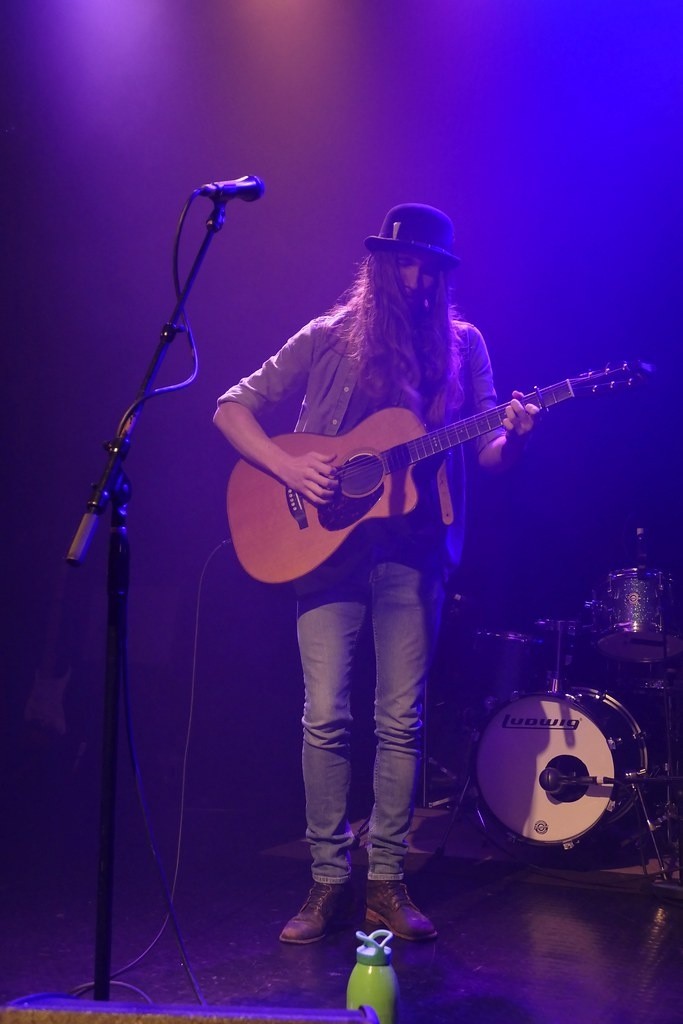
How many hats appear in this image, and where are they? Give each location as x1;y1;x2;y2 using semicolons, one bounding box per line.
363;203;459;266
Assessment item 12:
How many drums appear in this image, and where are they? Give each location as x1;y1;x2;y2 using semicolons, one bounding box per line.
583;568;683;663
471;687;648;846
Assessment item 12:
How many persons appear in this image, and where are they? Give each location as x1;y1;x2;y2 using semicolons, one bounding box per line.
211;200;543;942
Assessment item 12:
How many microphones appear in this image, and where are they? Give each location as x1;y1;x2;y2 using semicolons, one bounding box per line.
200;175;265;202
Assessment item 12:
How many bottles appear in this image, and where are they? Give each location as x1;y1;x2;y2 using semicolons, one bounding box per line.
346;927;398;1023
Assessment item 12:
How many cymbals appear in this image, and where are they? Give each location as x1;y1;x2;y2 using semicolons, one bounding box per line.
470;629;530;646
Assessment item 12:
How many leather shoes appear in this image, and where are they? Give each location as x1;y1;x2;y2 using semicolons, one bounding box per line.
367;878;437;939
280;882;348;944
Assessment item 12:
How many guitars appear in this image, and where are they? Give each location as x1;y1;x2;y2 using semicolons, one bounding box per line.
226;359;645;584
